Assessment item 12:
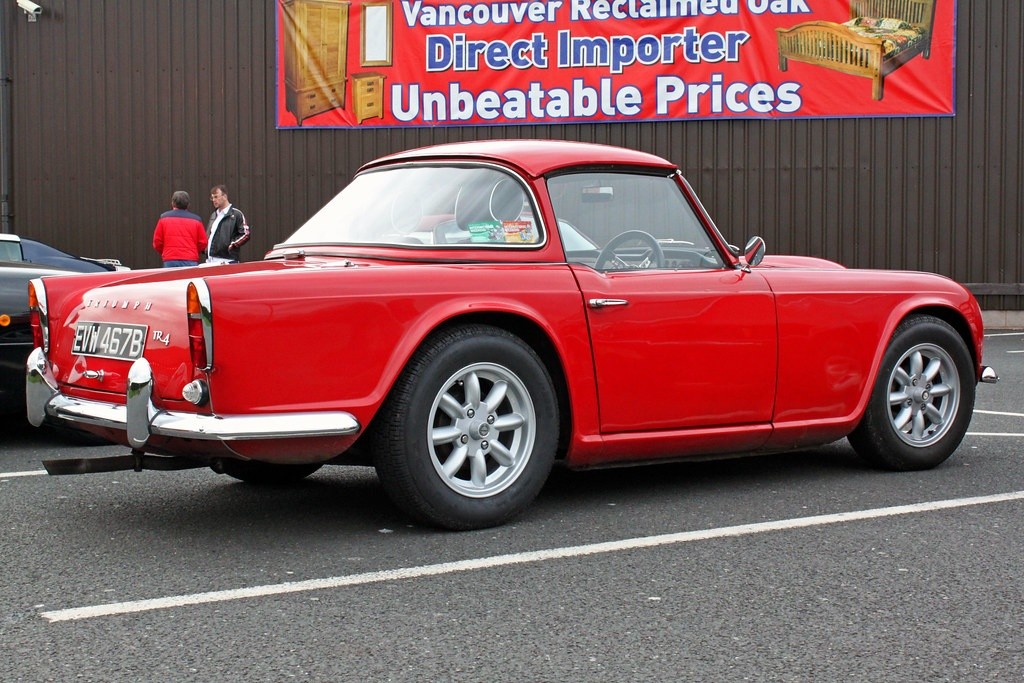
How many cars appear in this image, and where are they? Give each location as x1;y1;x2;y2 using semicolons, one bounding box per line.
0;234;130;427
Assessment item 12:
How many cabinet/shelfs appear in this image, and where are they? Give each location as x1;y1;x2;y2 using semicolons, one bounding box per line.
358;2;394;68
351;71;387;125
280;0;352;127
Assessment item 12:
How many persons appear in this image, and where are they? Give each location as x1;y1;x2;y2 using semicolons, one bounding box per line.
202;185;250;264
153;191;208;268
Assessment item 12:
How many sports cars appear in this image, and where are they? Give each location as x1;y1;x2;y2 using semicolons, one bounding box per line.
24;135;996;536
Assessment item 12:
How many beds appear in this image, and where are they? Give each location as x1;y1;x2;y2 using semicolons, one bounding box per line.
773;0;936;101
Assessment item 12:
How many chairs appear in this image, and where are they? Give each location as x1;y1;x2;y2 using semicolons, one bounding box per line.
367;188;424;244
454;178;525;244
